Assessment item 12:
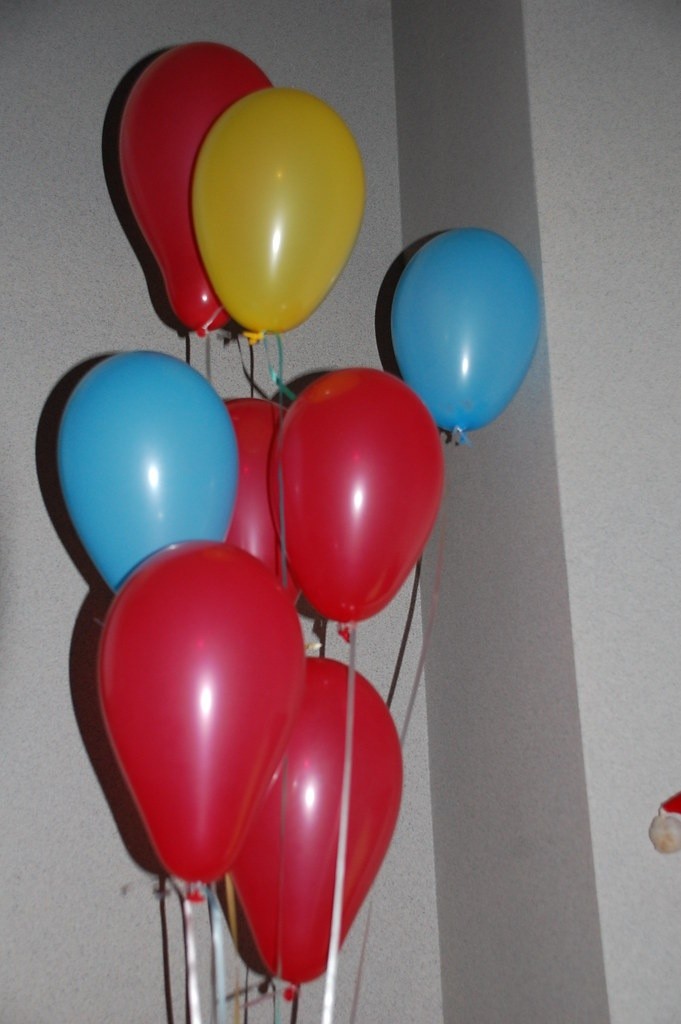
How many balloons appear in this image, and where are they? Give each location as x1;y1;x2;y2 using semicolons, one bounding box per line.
119;44;273;336
60;225;538;996
192;85;361;347
